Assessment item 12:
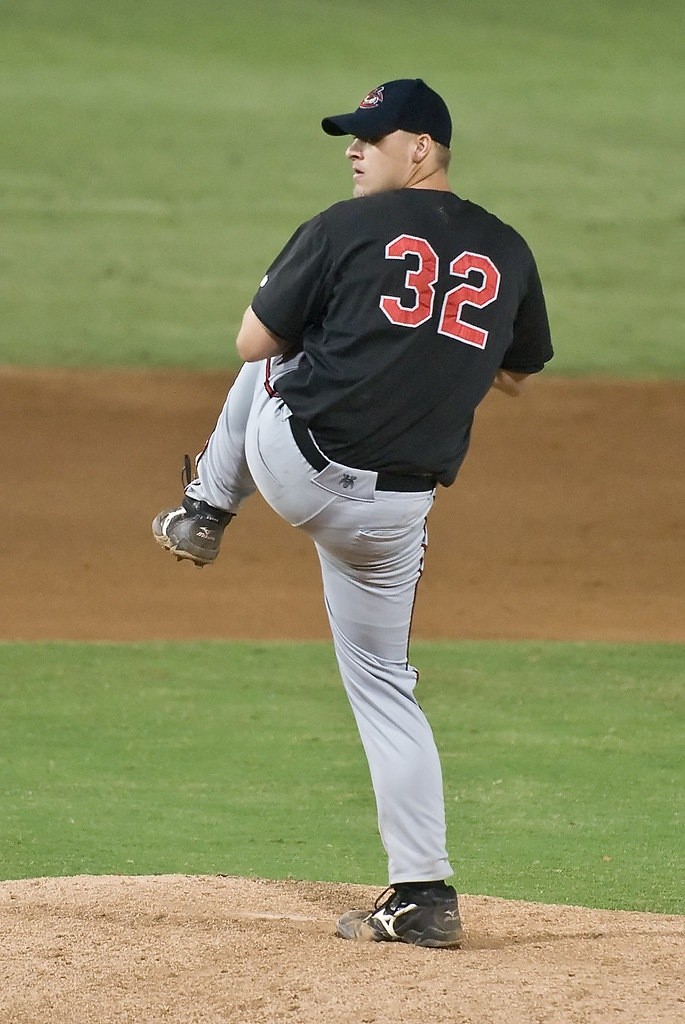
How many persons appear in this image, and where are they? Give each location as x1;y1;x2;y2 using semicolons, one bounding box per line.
150;79;554;951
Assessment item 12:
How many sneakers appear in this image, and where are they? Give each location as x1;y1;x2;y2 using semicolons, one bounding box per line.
336;885;464;947
152;454;237;566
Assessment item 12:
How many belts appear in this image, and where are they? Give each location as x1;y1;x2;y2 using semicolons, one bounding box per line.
288;415;437;492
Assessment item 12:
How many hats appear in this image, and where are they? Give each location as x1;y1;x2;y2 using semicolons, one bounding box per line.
321;78;452;148
